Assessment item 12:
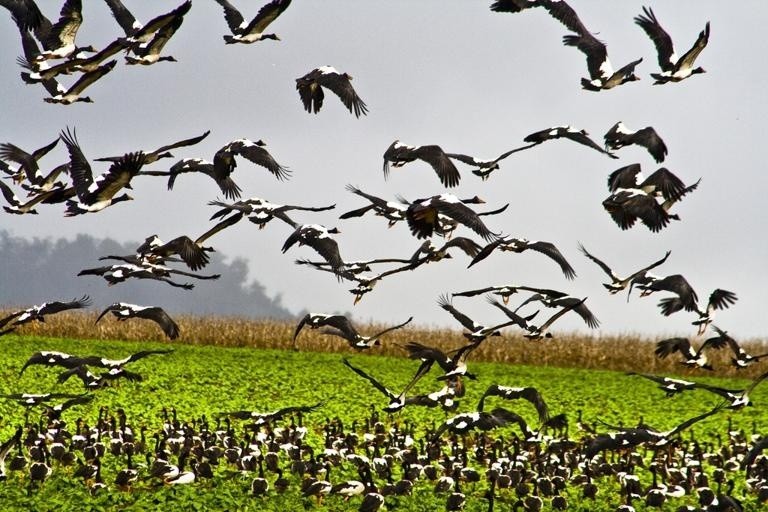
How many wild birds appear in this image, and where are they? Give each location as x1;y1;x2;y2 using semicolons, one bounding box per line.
562;34;643;92
0;122;768;512
490;0;587;38
635;5;711;85
0;0;193;105
216;1;290;44
295;64;370;118
